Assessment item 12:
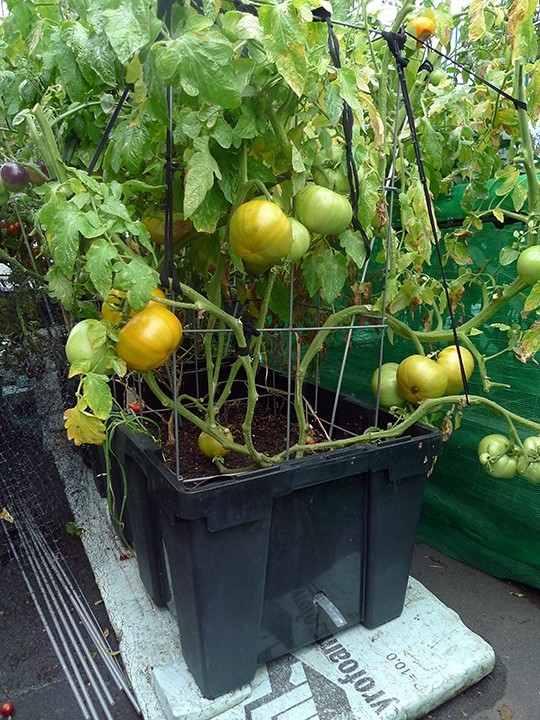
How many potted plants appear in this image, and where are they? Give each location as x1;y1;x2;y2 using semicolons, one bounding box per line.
0;0;540;700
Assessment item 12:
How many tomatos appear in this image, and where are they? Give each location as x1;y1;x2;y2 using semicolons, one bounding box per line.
0;161;50;255
406;16;444;85
65;144;540;485
